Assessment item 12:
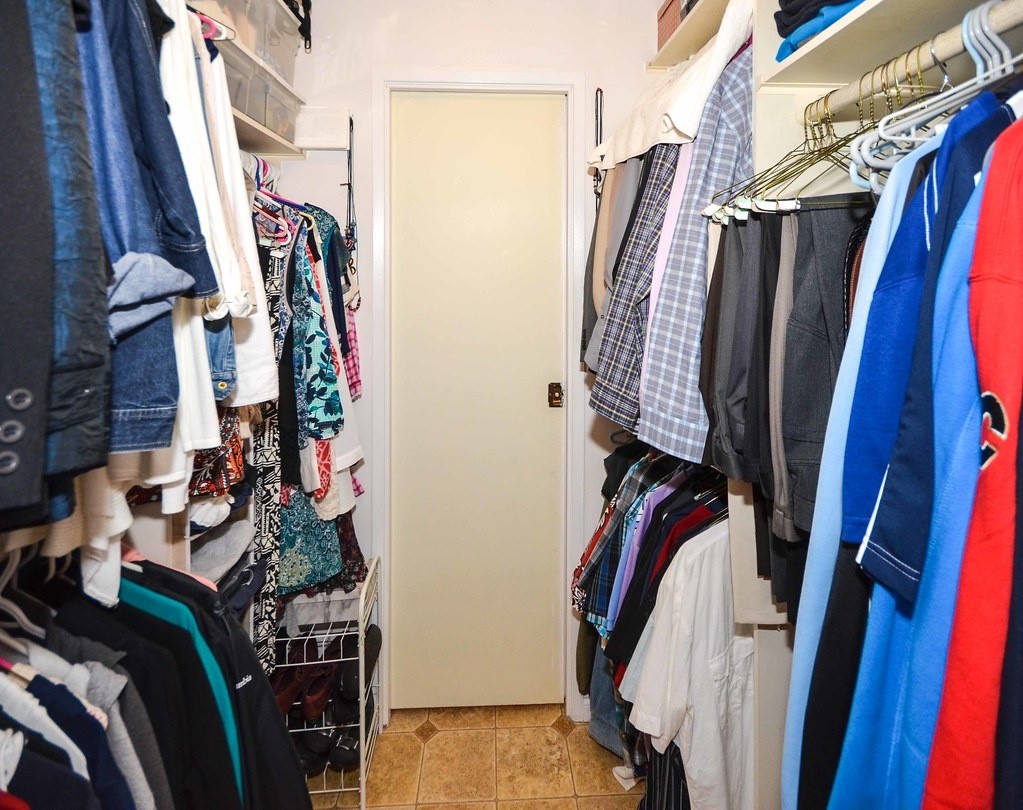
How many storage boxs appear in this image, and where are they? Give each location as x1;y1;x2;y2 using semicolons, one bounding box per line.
185;0;303;86
202;23;308;144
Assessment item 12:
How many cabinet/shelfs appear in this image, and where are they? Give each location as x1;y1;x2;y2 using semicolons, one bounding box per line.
267;556;386;810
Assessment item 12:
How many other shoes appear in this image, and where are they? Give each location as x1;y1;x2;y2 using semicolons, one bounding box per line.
271;632;318;715
339;624;382;700
301;630;350;720
271;683;373;770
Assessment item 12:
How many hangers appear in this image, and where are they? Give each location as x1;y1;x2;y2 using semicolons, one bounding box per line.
187;4;237;41
0;530;142;680
240;147;315;246
700;0;1023;226
609;430;729;527
